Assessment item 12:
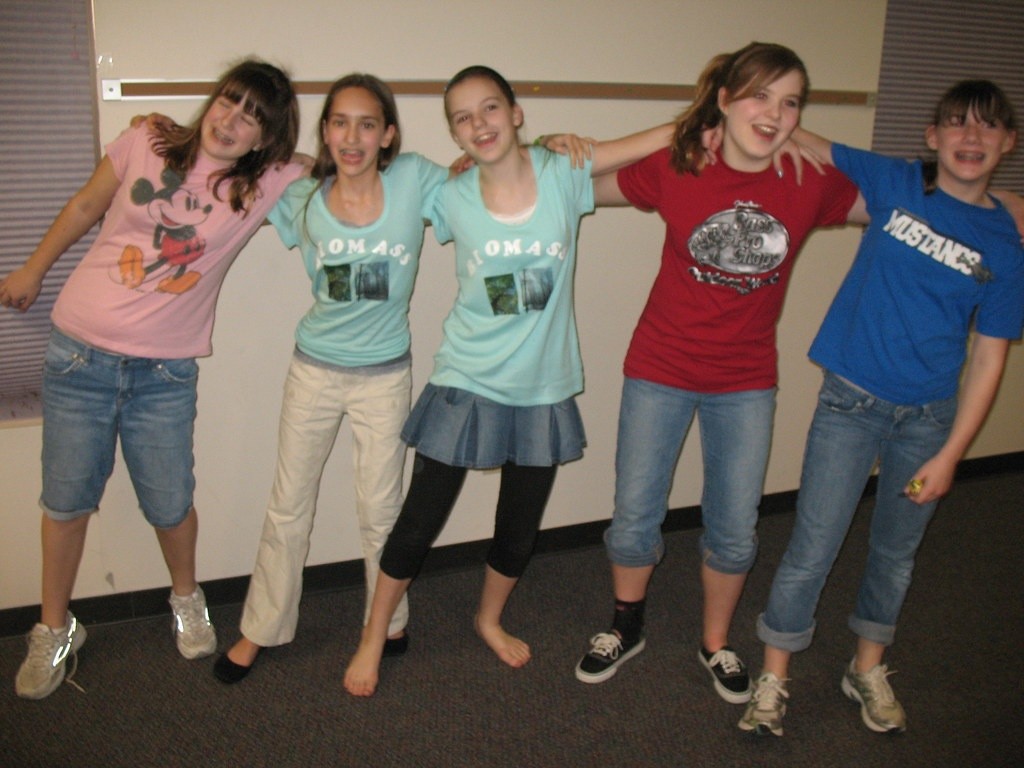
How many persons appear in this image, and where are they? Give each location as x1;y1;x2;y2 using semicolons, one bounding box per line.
0;54;466;699
342;65;829;696
692;80;1024;737
130;72;600;686
446;43;1024;704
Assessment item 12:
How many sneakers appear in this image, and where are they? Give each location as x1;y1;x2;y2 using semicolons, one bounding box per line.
842;658;907;734
168;588;215;660
16;611;87;699
575;631;645;682
737;677;789;738
699;646;752;705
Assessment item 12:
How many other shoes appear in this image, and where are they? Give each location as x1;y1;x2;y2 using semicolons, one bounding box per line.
214;654;248;683
382;631;407;657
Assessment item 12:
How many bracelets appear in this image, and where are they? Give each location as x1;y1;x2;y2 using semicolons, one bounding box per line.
533;135;544;146
